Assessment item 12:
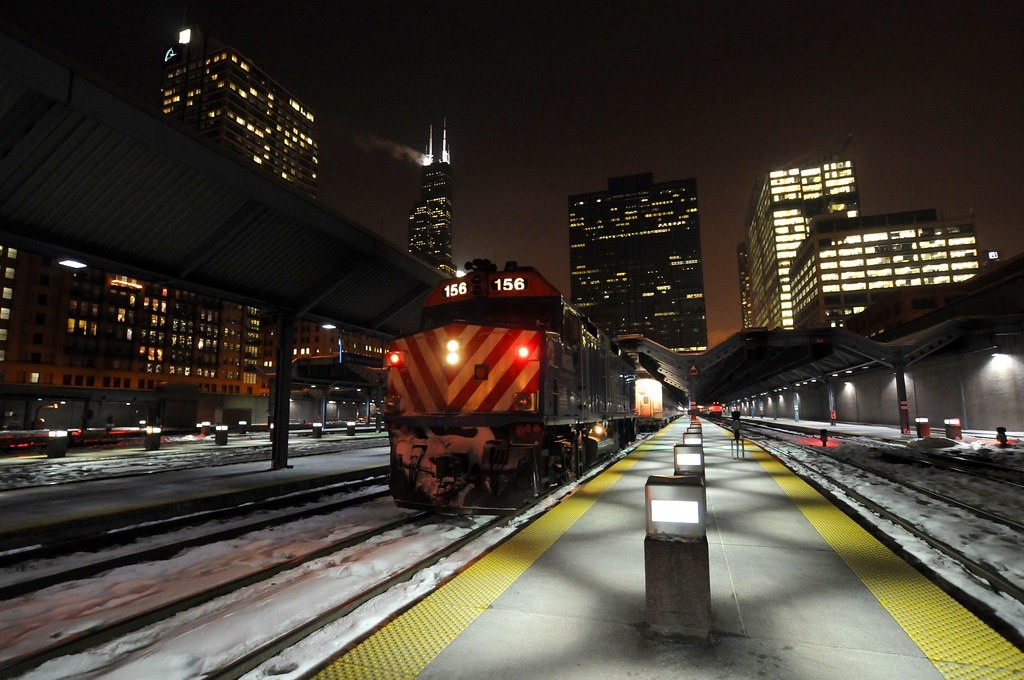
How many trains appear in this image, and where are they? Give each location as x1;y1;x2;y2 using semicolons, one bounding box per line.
382;264;687;514
